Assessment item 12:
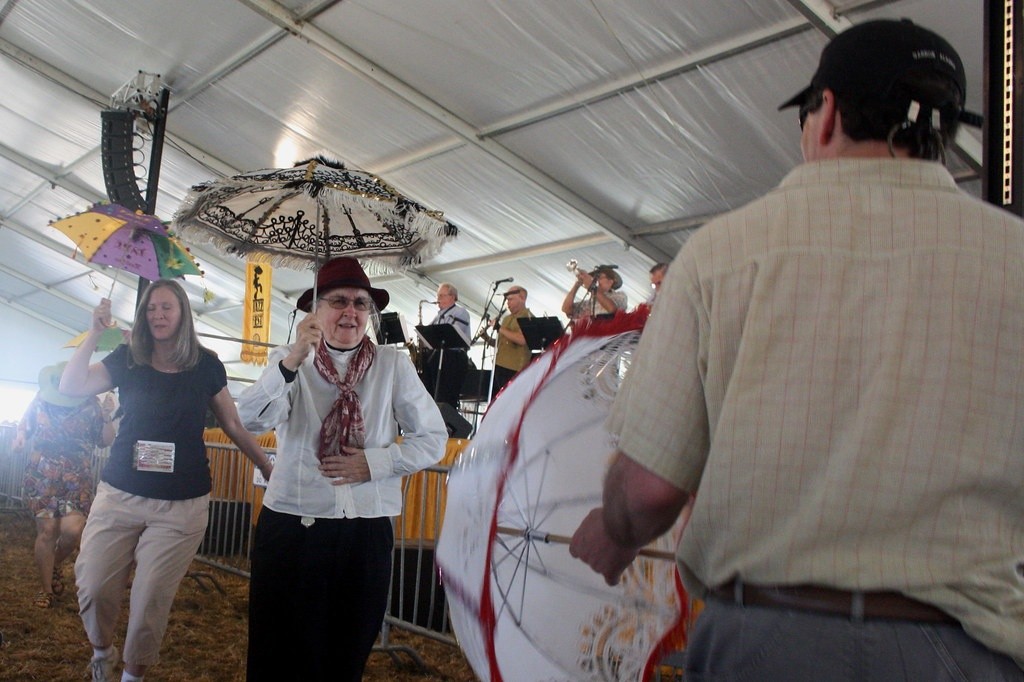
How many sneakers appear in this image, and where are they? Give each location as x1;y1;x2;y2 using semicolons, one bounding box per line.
85;645;121;682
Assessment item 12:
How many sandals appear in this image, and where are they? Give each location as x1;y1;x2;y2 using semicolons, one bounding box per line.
50;564;65;595
33;590;55;611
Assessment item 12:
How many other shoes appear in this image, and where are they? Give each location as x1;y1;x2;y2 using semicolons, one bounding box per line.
451;425;474;440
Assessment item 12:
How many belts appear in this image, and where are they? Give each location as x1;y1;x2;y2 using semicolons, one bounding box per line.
716;581;960;625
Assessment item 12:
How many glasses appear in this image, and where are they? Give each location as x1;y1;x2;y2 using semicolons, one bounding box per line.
435;294;451;300
318;293;374;311
650;281;661;288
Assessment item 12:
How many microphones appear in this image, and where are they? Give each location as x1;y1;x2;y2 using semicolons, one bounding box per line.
495;277;513;283
449;315;468;325
291;309;298;313
503;290;520;296
598;265;618;269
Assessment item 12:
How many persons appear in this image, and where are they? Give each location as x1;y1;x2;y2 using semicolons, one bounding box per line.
562;265;627;322
426;283;472;352
11;360;117;608
647;262;668;306
233;257;449;682
568;15;1023;682
480;285;536;406
58;279;274;682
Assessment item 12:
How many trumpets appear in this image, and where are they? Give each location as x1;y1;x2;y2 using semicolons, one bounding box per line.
565;259;584;287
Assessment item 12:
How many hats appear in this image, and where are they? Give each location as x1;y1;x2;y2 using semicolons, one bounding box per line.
777;17;967;123
588;264;624;290
297;255;390;315
37;361;91;408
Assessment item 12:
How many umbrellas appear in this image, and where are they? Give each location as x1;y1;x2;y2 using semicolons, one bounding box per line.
172;155;462;369
436;302;693;679
48;201;206;327
62;322;134;412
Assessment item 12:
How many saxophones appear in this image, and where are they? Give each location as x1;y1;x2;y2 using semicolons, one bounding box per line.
402;299;441;375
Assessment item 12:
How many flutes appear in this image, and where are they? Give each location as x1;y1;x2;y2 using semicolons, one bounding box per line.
468;307;508;346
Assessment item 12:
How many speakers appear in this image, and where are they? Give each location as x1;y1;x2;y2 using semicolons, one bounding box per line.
100;108;147;212
435;402;473;439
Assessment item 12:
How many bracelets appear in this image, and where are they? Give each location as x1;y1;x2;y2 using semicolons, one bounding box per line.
102;415;112;424
